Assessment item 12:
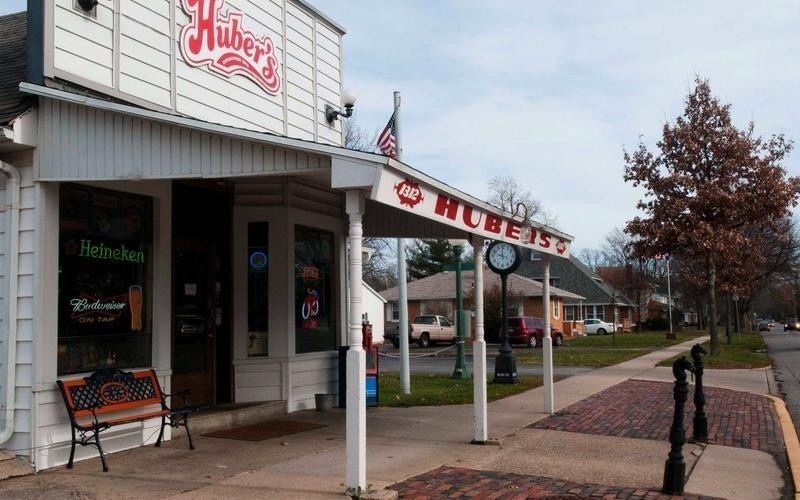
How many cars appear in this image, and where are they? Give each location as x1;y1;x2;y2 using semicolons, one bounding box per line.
583;319;617;335
754;318;775;333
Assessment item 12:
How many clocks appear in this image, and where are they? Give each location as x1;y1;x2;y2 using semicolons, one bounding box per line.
485;240;522;384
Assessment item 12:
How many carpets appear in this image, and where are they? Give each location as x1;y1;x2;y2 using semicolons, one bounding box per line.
199;419;330;440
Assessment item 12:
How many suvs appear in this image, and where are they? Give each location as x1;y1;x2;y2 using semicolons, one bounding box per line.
498;316;563;348
784;318;800;332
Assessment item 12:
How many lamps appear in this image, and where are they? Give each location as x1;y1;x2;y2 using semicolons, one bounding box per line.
325;89;357;123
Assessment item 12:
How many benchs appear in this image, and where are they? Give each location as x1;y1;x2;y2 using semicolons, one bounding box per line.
56;369;199;472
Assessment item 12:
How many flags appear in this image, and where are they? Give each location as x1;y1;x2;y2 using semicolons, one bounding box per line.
377;108;396;158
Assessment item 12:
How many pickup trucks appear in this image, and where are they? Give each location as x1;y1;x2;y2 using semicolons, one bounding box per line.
383;314;458;348
